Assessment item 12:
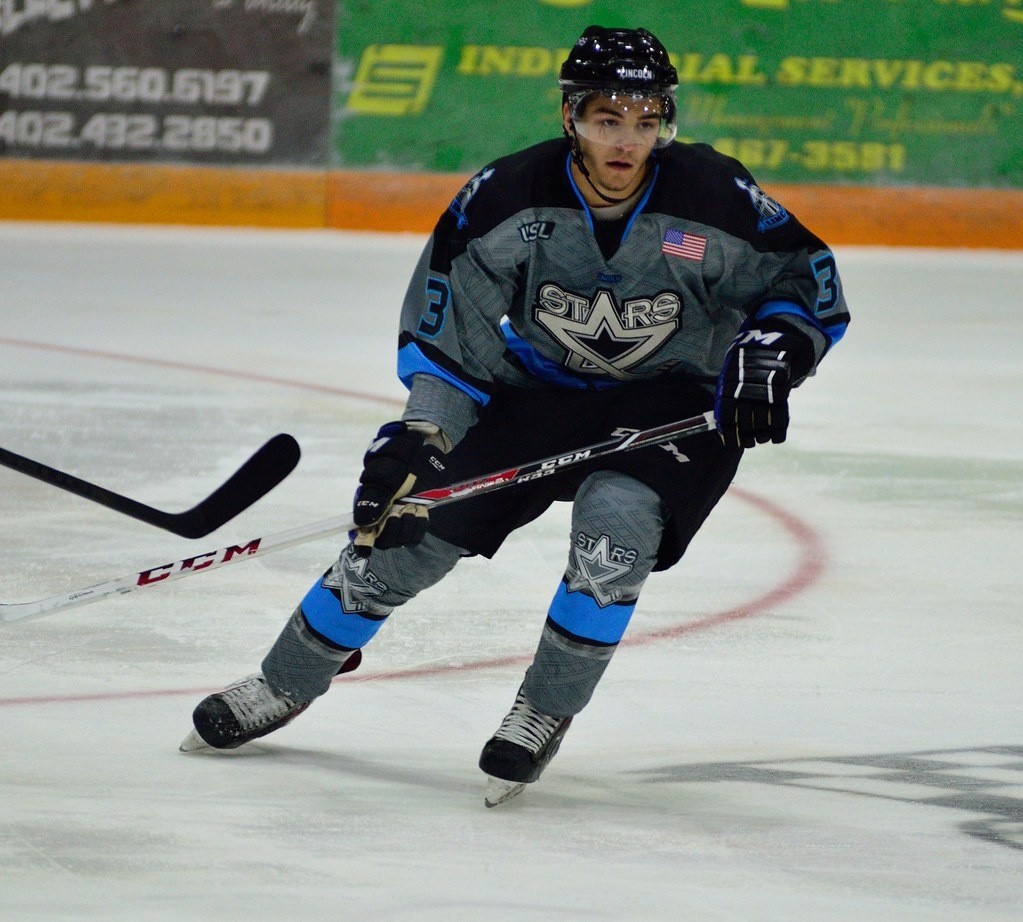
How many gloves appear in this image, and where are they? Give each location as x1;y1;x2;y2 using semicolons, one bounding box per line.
354;418;449;549
713;313;816;450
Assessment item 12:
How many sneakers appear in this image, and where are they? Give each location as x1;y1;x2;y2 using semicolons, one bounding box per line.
479;677;574;809
178;648;362;753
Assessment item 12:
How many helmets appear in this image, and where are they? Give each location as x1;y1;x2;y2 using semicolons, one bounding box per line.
556;23;679;95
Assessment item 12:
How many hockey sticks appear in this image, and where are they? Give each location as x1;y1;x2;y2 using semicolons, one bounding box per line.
1;430;305;541
0;408;718;633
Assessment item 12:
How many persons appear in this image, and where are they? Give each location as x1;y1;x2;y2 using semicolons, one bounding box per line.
180;22;851;807
